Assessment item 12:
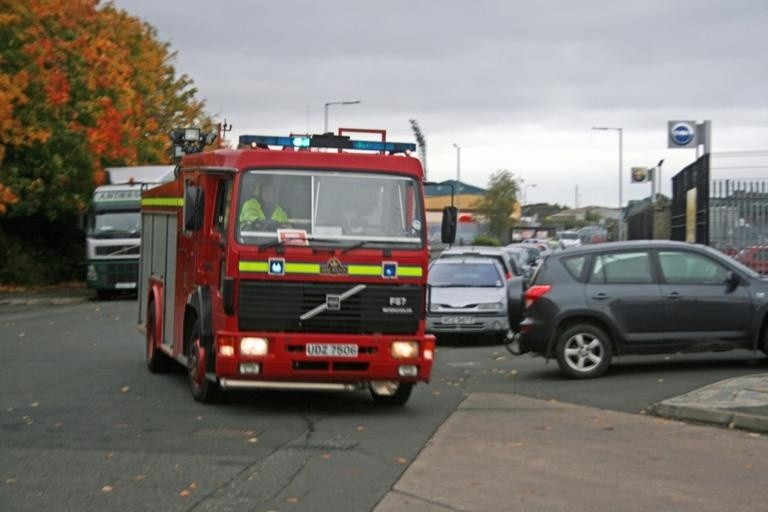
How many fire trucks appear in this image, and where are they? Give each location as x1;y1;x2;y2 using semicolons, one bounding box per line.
138;123;437;407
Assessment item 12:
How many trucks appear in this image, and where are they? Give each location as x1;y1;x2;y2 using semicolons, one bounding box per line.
76;164;175;300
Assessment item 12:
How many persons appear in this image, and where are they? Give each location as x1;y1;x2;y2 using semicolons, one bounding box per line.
340;183;402;236
239;180;290;231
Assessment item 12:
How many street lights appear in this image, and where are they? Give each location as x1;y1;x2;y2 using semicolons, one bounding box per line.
453;142;461;208
324;100;361;133
592;127;623;241
513;178;537;207
